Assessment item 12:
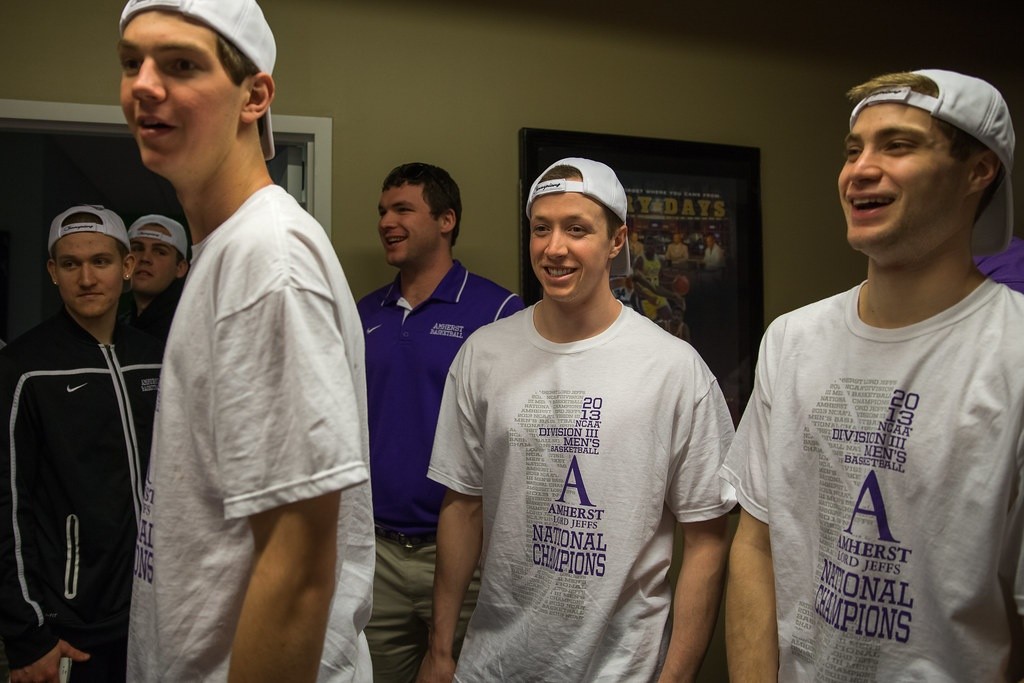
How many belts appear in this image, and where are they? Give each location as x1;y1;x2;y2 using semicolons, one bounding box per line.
374;527;437;547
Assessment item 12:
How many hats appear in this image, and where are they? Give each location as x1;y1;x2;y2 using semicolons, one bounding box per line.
128;214;188;259
119;0;276;161
848;69;1015;257
47;203;132;294
526;156;630;277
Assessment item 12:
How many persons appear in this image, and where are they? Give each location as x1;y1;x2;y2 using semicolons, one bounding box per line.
720;69;1024;683
117;0;377;683
354;161;528;683
972;236;1023;291
612;223;725;341
0;204;161;683
129;214;189;346
418;158;737;683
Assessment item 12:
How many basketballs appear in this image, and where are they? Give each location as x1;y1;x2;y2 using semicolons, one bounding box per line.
673;276;690;295
